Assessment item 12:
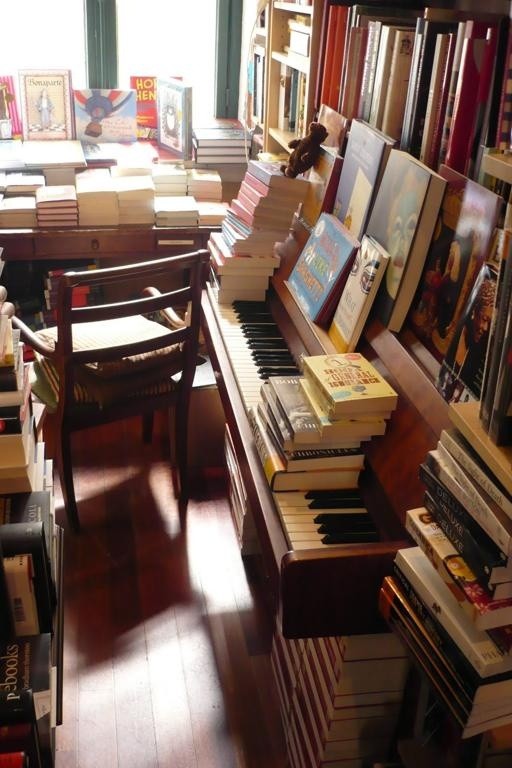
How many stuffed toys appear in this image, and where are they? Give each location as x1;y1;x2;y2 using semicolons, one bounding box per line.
279;122;329;180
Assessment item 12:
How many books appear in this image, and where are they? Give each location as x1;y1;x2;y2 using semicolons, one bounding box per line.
297;146;343;227
240;1;268;131
314;102;351;149
17;69;73;142
131;76;183;142
0;246;57;768
192;127;250;165
282;14;314;69
440;264;500;401
276;0;314;8
222;419;267;562
23;140;88;170
407;161;502;360
325;236;392;353
311;2;512;197
206;153;310;303
0;167;231;228
83;142;160;163
367;148;446;337
247;351;399;493
196;119;237;130
285;212;360;324
75;88;137;144
269;600;412;768
37;263;97;328
277;64;306;140
332;118;397;241
156;78;192;162
480;206;512;453
378;401;512;741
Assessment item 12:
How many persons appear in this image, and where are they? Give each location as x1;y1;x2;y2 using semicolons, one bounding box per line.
453;279;495;375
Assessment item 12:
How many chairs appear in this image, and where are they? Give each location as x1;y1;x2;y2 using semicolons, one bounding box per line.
11;250;211;531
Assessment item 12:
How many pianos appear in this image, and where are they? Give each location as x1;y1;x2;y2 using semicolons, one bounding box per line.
205;281;442;643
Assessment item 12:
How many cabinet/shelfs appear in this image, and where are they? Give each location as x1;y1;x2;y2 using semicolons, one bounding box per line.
235;0;326;166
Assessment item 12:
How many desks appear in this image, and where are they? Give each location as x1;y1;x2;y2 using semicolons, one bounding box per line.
0;224;223;304
